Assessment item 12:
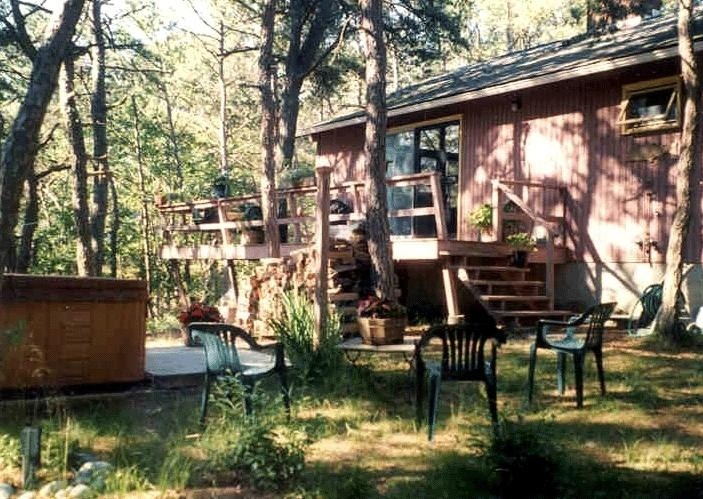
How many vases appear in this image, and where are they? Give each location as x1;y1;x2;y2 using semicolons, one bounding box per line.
358;317;405;345
181;323;204;348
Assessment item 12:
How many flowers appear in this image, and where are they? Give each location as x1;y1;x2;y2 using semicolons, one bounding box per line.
177;302;224;326
355;293;407;319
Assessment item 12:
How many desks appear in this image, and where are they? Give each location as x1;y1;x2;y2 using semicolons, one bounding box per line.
336;341;416;372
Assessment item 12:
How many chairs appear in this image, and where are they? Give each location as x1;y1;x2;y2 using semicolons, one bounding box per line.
184;316;293;431
408;295;620;440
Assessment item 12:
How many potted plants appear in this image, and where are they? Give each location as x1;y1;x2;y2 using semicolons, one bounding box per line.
469;197;538;270
